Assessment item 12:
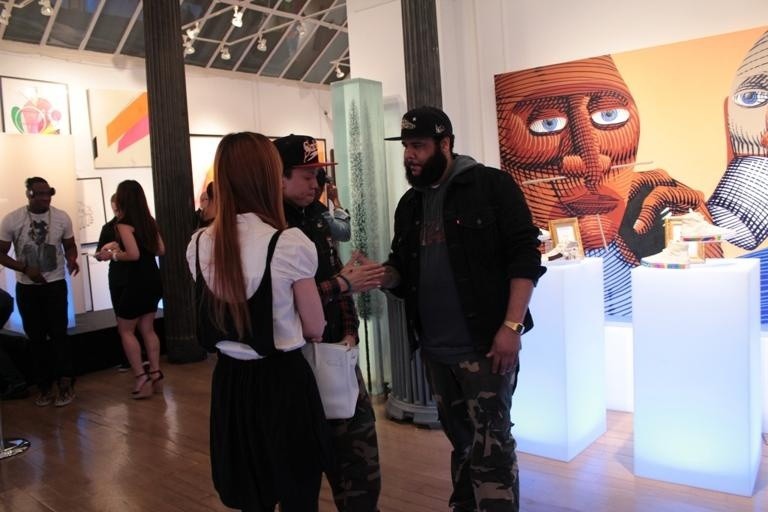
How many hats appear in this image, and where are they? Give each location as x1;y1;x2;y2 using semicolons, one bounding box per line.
272;134;337;168
384;106;452;140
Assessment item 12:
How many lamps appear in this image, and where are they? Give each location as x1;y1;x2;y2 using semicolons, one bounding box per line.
256;35;267;51
334;65;344;79
220;47;231;60
232;5;245;28
182;34;196;59
186;22;200;41
296;20;309;37
39;0;54;16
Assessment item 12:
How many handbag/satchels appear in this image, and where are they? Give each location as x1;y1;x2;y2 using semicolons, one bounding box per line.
301;342;360;420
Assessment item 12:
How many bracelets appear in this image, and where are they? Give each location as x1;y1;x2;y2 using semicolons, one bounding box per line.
112;252;118;263
22;264;29;273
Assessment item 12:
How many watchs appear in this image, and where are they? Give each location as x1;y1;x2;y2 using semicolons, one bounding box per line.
503;320;525;336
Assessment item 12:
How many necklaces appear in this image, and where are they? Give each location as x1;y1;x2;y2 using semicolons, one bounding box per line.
28;206;50;237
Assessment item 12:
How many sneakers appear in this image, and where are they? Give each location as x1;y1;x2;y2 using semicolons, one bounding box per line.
54;391;76;407
35;392;55;406
142;353;150;367
117;365;130;372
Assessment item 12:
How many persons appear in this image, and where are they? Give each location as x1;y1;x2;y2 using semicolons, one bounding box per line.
101;179;166;399
353;105;548;511
0;175;80;408
95;192;151;373
185;131;384;511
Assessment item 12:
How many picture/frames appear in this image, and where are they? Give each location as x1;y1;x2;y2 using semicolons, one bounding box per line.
190;133;225;211
76;177;108;246
548;217;585;260
82;252;94;312
664;215;705;264
267;136;329;208
0;75;72;134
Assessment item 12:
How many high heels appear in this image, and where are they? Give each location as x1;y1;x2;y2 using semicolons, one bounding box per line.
128;372;152;399
147;368;164;392
